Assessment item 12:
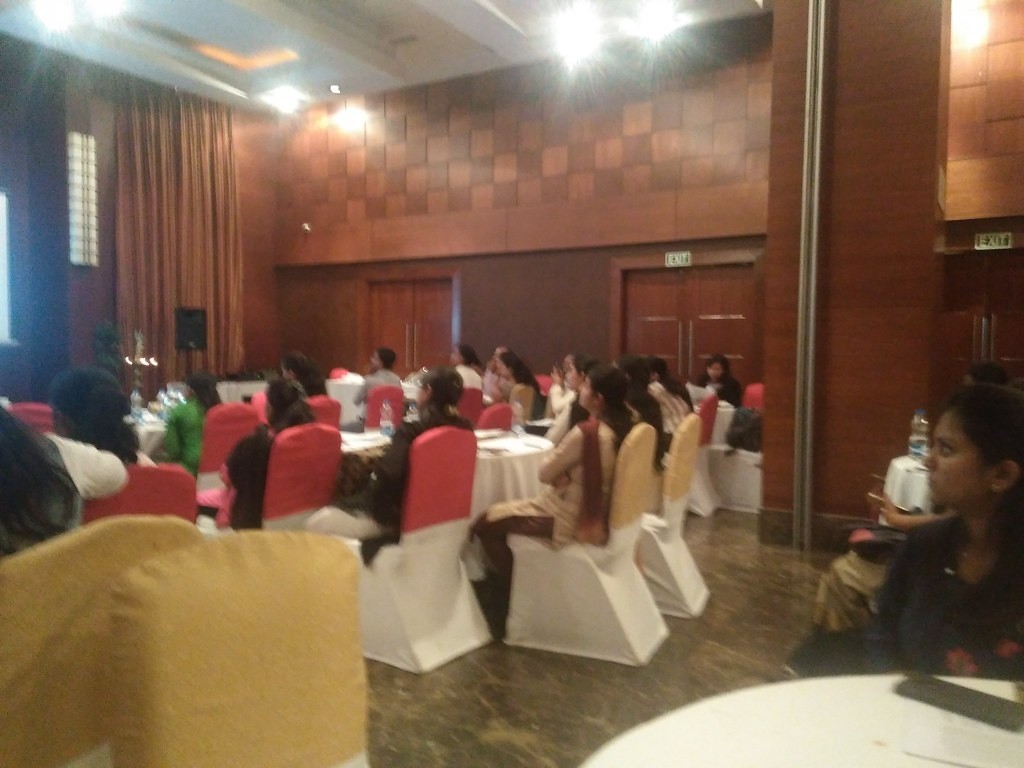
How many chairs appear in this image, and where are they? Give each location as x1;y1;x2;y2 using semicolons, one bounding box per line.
0;367;766;768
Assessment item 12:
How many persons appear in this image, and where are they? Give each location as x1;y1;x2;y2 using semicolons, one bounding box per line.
786;362;1024;681
222;376;351;531
470;346;744;620
360;363;473;563
153;368;222;480
338;349;404;433
50;366;161;468
447;344;483;417
280;348;329;394
0;395;129;558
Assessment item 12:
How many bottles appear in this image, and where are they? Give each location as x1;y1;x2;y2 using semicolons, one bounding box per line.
909;408;929;462
379;401;393;435
510;395;527;442
130;389;142;420
156;387;166;421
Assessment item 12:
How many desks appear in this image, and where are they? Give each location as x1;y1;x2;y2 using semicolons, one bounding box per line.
693;400;736;477
879;454;932;528
580;674;1024;768
341;430;556;580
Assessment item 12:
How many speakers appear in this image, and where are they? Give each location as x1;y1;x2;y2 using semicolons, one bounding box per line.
174;307;206;350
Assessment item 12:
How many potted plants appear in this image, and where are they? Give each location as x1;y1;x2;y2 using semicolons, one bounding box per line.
713;408;762;514
